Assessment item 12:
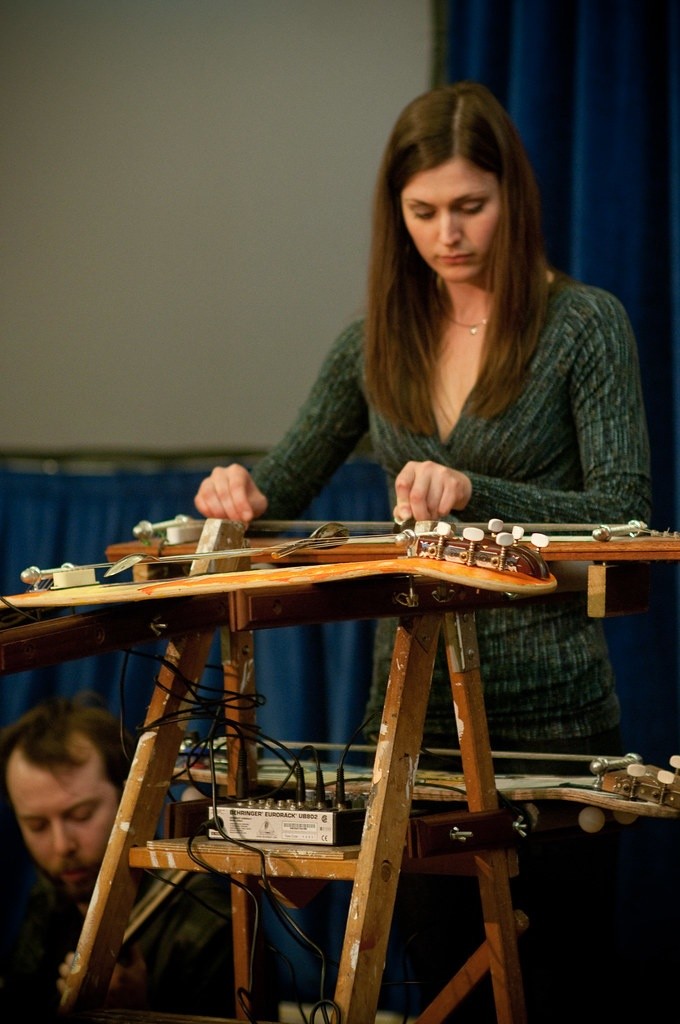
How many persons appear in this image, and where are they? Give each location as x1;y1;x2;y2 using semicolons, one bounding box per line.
0;696;316;1024
194;83;651;1024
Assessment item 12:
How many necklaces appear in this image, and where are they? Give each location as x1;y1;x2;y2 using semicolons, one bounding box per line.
436;302;488;334
